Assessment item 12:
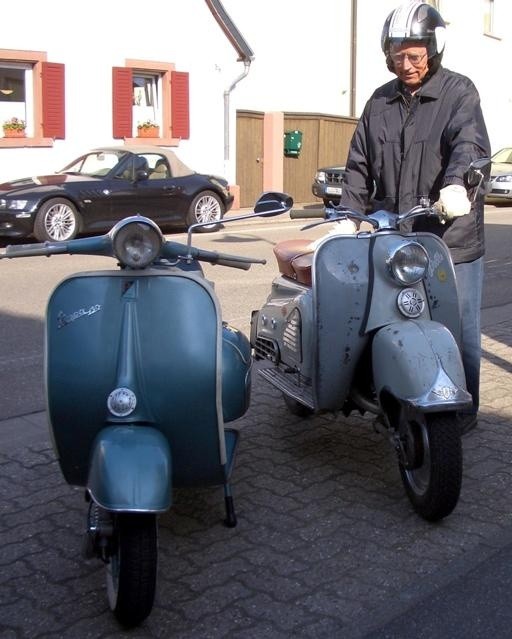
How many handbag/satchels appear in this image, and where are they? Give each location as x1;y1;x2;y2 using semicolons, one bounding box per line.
272;239;312;287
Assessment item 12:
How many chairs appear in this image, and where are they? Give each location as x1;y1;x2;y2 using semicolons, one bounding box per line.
94;157;169;182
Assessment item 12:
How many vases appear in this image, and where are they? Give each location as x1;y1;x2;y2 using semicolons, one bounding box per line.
4;128;25;137
138;127;159;137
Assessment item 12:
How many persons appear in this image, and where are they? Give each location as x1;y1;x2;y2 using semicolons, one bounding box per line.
305;3;491;435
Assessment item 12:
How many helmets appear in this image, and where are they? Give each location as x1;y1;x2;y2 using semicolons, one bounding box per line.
381;2;446;75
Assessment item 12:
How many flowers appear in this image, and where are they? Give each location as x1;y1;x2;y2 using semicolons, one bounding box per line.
1;118;24;129
137;120;160;128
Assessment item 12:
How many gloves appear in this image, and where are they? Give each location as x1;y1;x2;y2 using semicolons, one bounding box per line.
437;184;471;224
305;219;358;250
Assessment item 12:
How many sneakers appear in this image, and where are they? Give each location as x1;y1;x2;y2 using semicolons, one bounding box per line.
456;413;478;435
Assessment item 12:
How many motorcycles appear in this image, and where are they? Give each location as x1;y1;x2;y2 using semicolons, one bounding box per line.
0;188;298;629
247;155;493;523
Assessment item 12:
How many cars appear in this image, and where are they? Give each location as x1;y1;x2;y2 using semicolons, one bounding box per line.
1;143;237;245
309;164;349;209
482;146;512;208
